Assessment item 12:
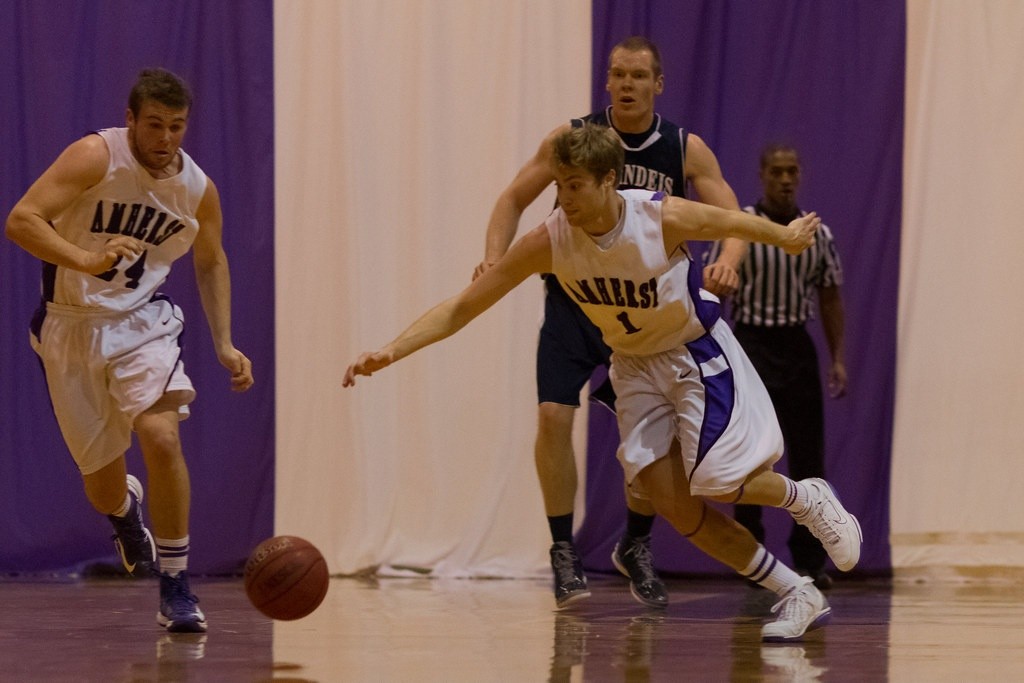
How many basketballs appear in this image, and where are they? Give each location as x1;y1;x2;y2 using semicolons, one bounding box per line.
244;534;331;621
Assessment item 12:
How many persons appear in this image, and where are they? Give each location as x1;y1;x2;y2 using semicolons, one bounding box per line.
4;66;255;633
342;37;864;642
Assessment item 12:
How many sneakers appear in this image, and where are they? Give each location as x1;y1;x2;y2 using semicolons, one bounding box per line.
148;566;208;632
789;477;864;573
554;610;587;667
611;533;671;609
759;643;829;683
796;569;833;591
107;473;157;576
623;612;664;671
745;578;768;590
760;575;832;642
549;541;592;608
